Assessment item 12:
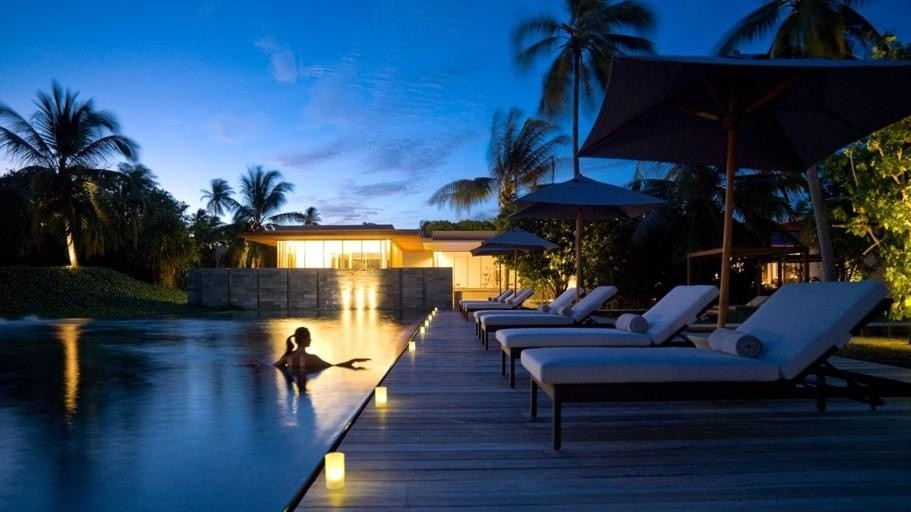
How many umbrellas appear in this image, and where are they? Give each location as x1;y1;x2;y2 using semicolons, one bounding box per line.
575;49;911;328
469;225;561;298
508;174;669;304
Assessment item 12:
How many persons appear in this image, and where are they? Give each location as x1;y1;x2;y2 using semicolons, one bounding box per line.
245;327;371;368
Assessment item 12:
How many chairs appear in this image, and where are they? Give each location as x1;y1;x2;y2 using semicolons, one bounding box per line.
520;281;890;454
459;288;536;321
473;287;584;340
494;282;720;389
480;286;618;352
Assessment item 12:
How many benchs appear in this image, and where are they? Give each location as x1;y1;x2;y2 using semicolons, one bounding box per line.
861;320;910;340
